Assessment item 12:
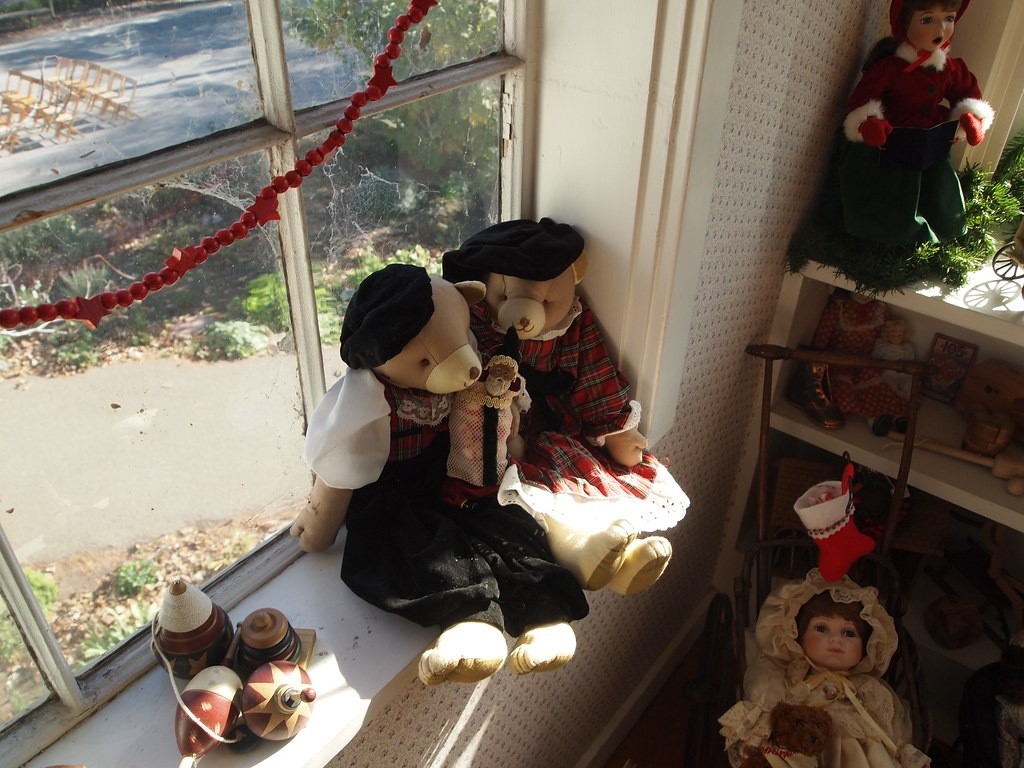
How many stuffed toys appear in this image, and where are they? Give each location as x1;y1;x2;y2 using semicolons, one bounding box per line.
443;217;689;595
292;265;589;684
739;701;832;768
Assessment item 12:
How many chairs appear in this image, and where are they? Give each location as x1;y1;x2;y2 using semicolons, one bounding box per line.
0;55;143;161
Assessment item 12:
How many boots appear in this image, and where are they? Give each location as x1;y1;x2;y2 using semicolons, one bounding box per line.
784;343;844;432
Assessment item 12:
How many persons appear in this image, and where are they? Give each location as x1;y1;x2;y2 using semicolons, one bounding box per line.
874;320;915;399
720;568;932;768
810;291;910;435
806;0;995;273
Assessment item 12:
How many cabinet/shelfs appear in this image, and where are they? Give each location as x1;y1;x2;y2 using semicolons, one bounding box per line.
708;220;1024;758
